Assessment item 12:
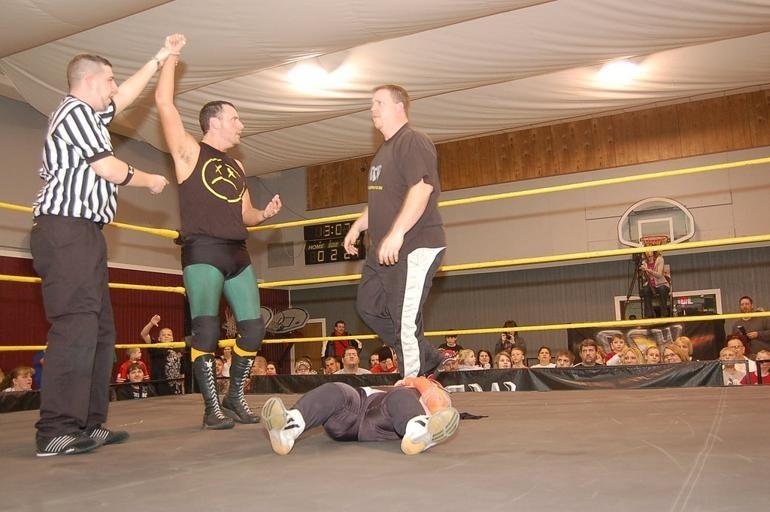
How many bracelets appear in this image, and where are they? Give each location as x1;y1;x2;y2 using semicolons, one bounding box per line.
155;56;161;69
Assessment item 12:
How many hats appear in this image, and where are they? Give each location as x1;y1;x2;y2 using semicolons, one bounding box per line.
441;349;459;365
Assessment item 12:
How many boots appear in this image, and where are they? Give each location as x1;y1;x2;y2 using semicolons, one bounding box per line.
221;347;260;423
194;354;234;429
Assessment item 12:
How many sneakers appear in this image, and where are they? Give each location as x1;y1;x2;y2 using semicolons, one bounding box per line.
262;397;298;455
400;406;460;455
34;433;100;456
84;425;128;445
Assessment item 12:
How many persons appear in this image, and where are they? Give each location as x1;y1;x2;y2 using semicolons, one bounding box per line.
342;84;449;398
116;315;187;398
294;319;400;375
719;337;770;386
212;345;277;378
258;372;461;458
0;314;51;391
731;296;770;352
435;320;528;372
29;32;187;458
531;336;700;369
155;32;283;429
638;244;672;317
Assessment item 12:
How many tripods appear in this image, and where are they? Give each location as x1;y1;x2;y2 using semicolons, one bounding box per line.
622;263;664;320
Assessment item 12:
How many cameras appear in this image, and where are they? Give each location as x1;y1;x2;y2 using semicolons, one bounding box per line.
503;332;511;339
